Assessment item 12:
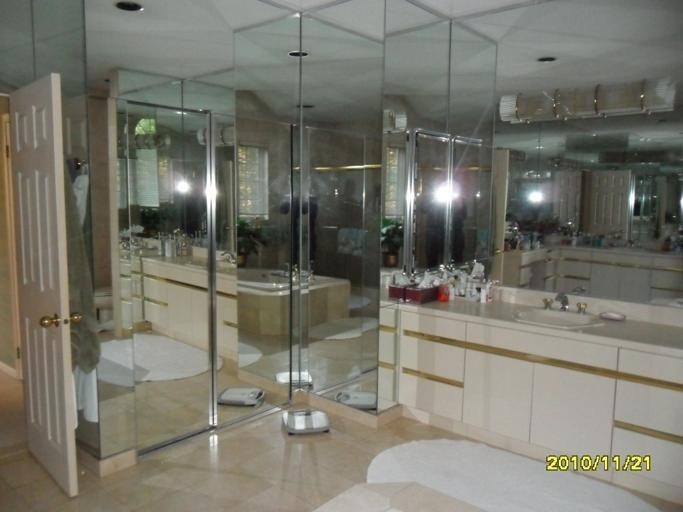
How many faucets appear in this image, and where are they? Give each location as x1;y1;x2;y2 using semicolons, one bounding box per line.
554;292;569;311
221;251;235;261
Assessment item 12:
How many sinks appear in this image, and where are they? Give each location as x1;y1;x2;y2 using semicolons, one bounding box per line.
511;307;604;329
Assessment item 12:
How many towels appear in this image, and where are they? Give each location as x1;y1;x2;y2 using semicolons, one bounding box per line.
74;365;99;423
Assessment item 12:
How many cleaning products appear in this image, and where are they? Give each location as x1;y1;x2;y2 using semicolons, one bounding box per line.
164;230;191;258
438;273;496;305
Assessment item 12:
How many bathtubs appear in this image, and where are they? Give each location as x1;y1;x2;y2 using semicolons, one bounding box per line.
238;267;289;293
